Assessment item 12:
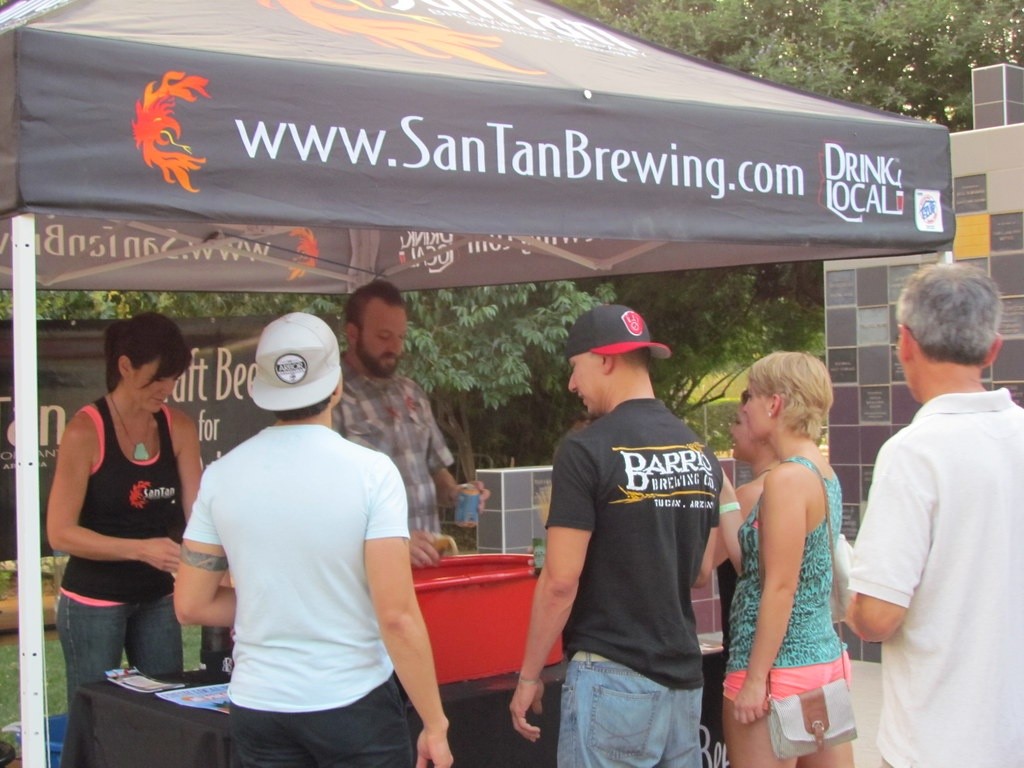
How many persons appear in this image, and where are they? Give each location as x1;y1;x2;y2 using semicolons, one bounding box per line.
174;313;454;768
842;262;1024;768
509;305;723;768
719;352;855;768
47;312;202;714
713;392;781;666
332;280;490;568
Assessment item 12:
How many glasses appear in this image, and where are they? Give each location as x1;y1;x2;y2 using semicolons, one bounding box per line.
740;389;752;405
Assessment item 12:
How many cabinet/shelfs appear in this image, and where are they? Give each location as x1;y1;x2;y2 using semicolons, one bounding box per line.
476;466;555;555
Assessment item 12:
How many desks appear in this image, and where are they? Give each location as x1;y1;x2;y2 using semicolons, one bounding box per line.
59;640;741;768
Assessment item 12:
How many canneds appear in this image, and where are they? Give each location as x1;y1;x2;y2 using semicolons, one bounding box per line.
454;484;481;528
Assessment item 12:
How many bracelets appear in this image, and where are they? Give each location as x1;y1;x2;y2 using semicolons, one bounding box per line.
719;503;740;514
518;679;538;684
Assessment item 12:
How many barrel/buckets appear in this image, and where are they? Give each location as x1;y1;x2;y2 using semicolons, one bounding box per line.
2;713;69;768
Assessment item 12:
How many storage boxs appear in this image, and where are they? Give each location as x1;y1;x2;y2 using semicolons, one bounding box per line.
410;552;563;682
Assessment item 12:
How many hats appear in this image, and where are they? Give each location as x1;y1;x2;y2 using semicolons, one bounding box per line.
565;304;675;362
248;312;345;415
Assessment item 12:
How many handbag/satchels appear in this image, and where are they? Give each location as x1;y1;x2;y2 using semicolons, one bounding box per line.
763;676;861;759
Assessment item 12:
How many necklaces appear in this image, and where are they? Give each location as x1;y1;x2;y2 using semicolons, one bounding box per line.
111;393;150;460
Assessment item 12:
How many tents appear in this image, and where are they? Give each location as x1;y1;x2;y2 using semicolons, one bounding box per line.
0;0;956;768
